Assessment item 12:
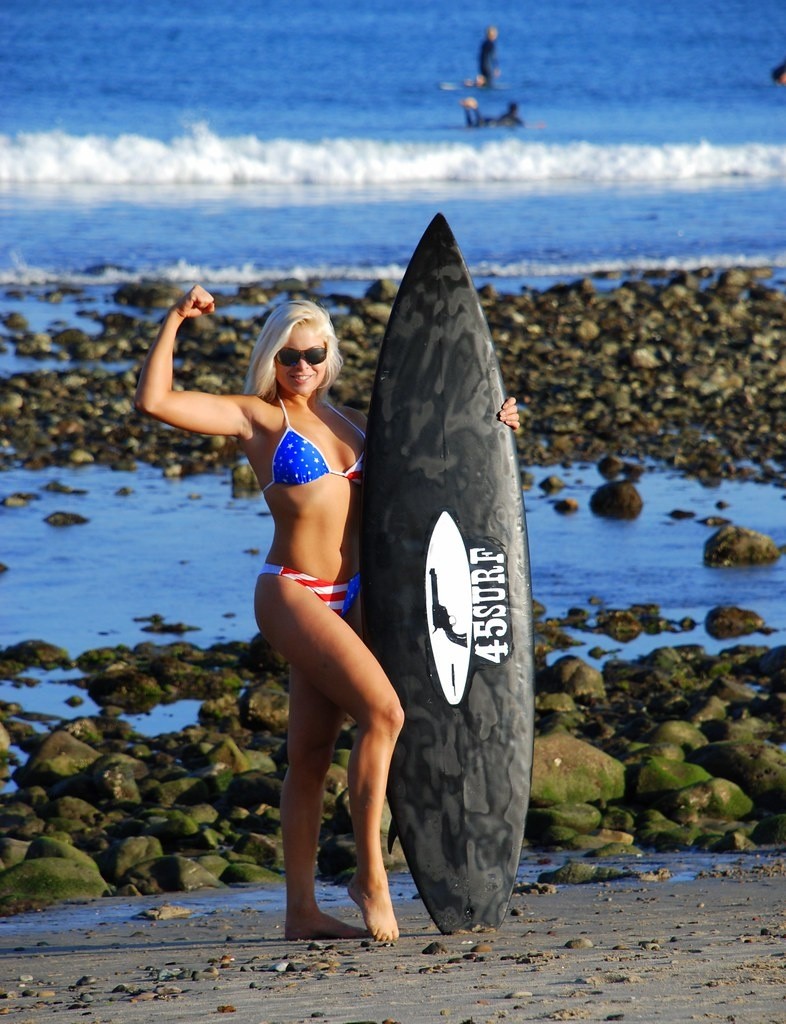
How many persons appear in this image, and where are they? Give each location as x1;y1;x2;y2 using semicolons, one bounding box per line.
135;284;520;943
461;96;547;130
773;65;786;84
464;24;501;88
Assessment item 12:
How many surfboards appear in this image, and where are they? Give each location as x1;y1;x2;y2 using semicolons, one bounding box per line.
357;213;538;934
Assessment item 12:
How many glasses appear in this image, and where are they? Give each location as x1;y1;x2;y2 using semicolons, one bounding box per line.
277;347;326;367
